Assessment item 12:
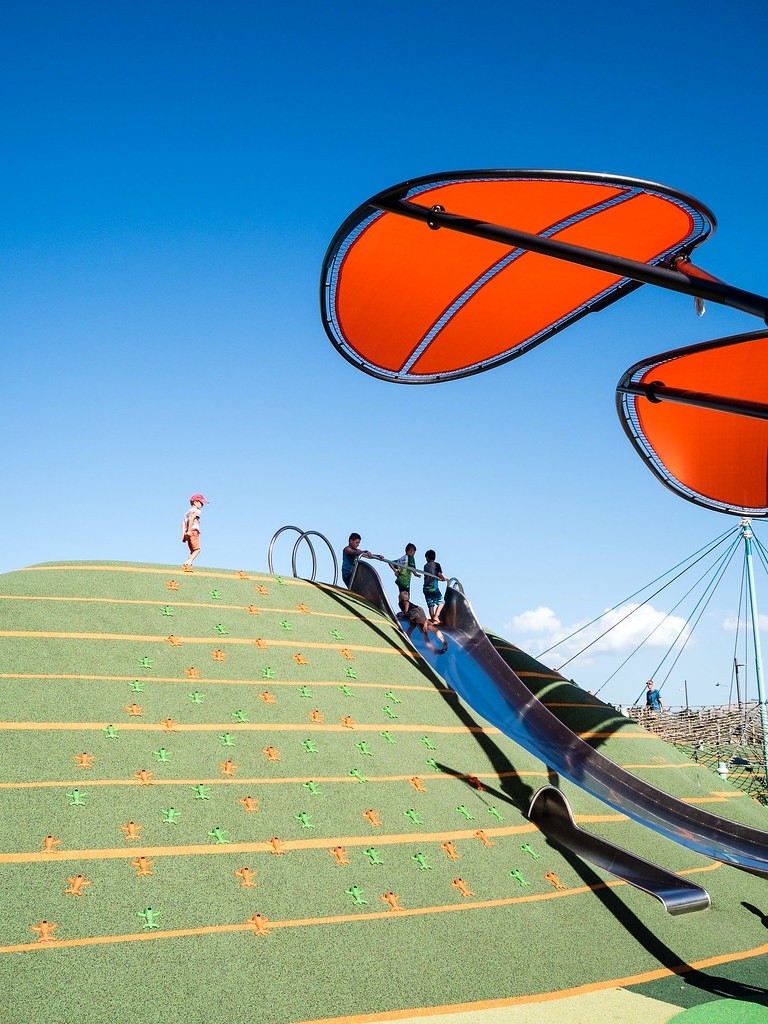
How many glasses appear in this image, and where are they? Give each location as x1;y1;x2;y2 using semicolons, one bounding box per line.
646;682;653;685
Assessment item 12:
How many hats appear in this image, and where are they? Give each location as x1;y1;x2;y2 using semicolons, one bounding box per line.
190;494;209;505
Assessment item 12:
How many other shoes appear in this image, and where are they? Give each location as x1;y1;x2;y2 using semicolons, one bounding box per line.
182;564;194;572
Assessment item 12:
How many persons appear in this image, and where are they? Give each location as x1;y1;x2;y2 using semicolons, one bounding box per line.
646;680;665;714
182;494;209;572
342;533;384;589
388;543;421;600
398;591;447;651
423;550;445;624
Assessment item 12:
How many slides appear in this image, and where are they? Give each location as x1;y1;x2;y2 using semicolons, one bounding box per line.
526;784;711;917
349;558;768;873
